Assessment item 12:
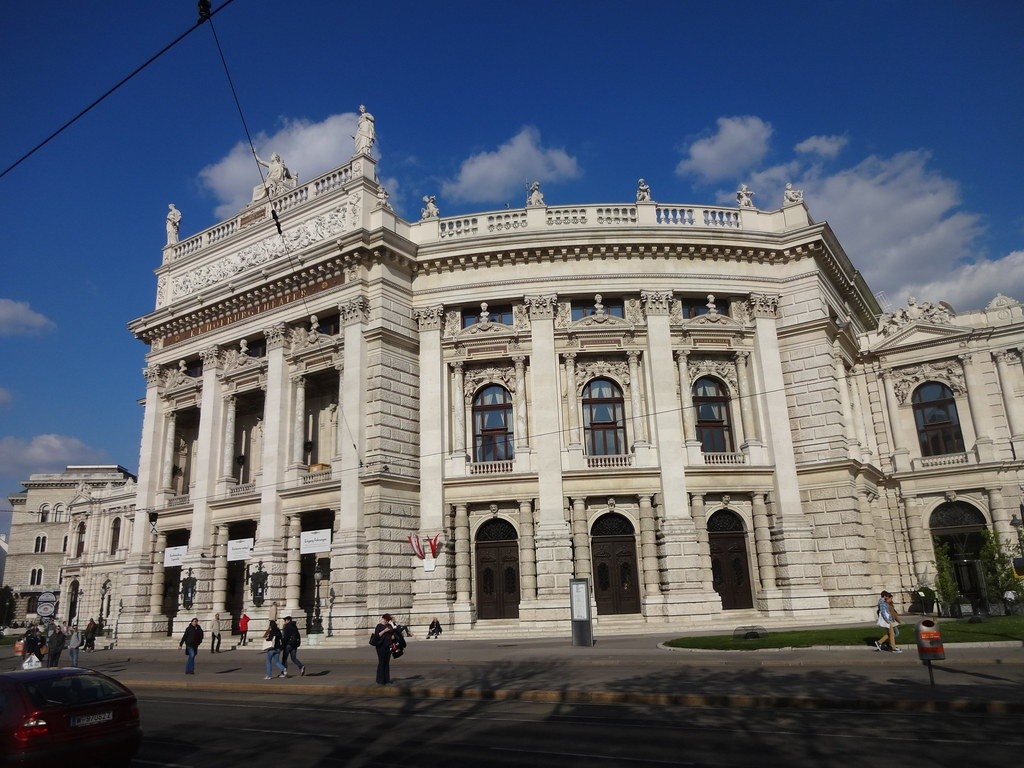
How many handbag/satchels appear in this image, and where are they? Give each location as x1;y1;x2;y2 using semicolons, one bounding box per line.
877;610;890;628
369;633;383;646
891;621;900;627
262;636;276;651
40;645;49;654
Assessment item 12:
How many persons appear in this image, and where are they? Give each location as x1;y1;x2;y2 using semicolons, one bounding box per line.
706;295;716;308
737;184;756;207
636;179;651;200
374;614;395;686
530;181;543;205
263;616;305;679
310;315;320;329
784;182;804;201
210;614;221;653
166;204;182;245
480;302;490;316
13;618;97;668
179;359;187;372
874;590;903;653
240;339;249;352
238;614;250;645
594;294;604;309
179;618;203;674
269;602;278;621
426;617;442;639
390;617;412;637
253;147;285;201
355;105;376;156
421;195;439;218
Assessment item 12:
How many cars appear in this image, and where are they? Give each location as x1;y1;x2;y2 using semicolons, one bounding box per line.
0;625;28;637
0;666;143;768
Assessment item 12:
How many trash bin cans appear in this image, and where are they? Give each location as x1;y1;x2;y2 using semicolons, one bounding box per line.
915;619;947;659
14;638;24;656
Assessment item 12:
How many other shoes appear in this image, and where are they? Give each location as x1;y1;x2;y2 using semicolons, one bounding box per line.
284;668;287;675
185;672;194;675
875;641;881;651
892;649;902;653
426;637;430;639
264;676;272;680
435;636;437;639
889;647;898;651
279;674;285;678
300;666;305;676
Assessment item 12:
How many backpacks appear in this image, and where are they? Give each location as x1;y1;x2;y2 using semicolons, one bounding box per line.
77;632;84;646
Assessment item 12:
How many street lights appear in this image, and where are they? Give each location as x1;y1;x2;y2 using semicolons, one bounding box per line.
95;586;106;636
308;561;325;634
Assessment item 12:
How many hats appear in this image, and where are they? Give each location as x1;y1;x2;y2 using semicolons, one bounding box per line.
382;614;392;620
